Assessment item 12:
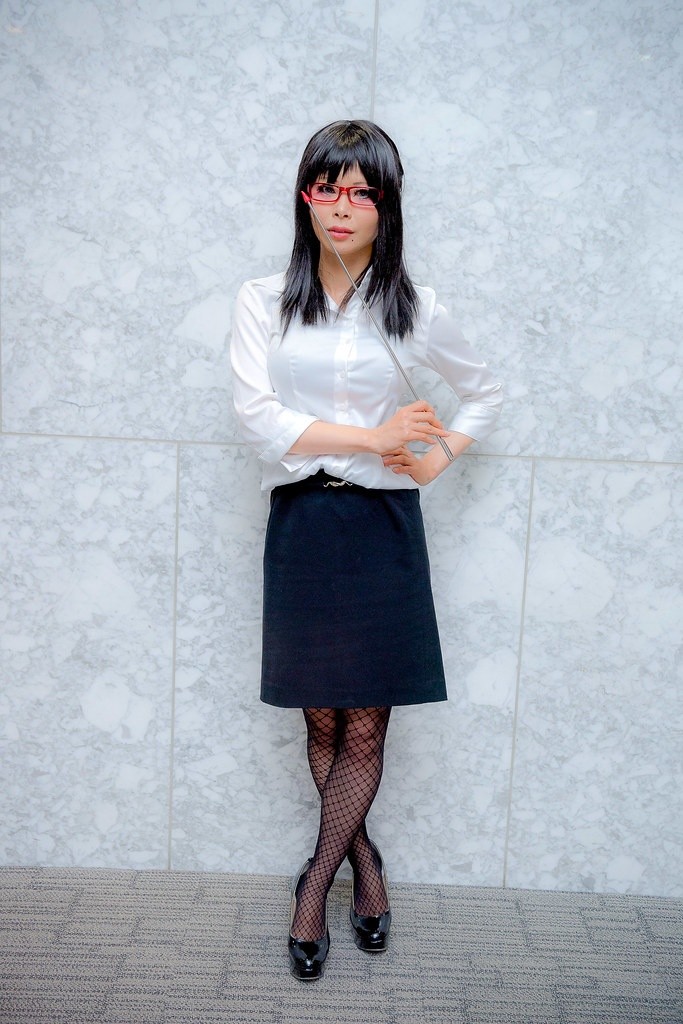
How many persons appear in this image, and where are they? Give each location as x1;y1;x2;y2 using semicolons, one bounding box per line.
229;119;505;983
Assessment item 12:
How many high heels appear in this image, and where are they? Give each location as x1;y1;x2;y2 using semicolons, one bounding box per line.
288;857;330;980
349;842;392;953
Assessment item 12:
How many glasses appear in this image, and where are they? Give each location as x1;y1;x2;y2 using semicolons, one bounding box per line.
308;181;384;207
